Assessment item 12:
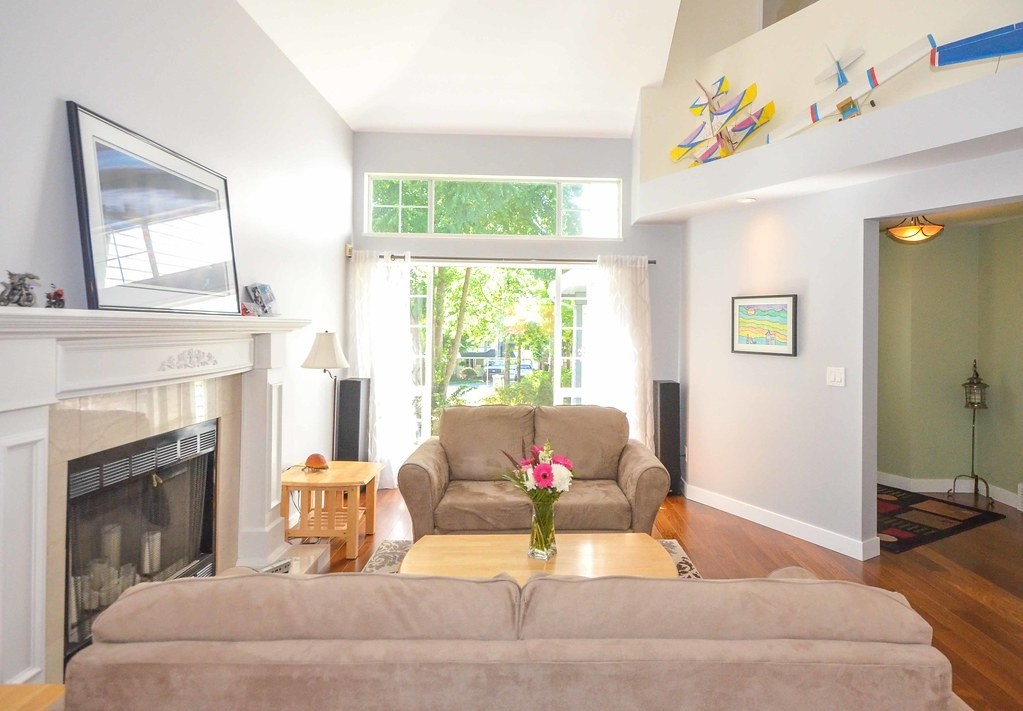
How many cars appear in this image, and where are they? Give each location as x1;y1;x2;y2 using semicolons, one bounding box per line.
504;366;517;381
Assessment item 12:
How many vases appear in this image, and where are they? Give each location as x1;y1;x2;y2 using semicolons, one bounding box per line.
521;493;564;561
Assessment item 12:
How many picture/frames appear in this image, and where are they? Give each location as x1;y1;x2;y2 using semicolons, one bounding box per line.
730;295;797;357
66;97;242;313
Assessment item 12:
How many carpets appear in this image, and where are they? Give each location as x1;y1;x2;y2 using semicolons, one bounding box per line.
877;484;1006;552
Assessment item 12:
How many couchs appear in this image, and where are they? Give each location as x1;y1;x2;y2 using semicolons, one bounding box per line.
64;576;975;710
397;404;672;550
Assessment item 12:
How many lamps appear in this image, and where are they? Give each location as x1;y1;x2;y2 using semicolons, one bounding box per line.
301;330;350;463
881;215;949;247
948;358;996;502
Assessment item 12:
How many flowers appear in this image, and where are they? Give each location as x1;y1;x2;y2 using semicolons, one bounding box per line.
505;438;576;551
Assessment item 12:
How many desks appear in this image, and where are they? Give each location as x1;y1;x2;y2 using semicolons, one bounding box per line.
282;453;385;556
398;529;677;581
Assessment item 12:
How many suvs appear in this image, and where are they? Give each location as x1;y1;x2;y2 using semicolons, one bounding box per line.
515;364;532;380
481;360;504;381
